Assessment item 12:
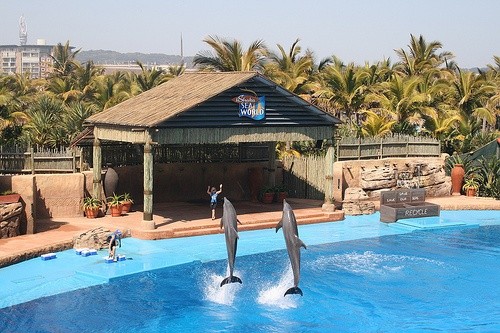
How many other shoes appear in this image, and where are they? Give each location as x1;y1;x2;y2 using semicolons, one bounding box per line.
212;216;217;220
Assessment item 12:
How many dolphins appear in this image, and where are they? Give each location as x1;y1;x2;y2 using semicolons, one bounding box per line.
219;196;243;288
275;198;308;298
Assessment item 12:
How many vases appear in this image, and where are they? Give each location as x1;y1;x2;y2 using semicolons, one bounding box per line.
102;163;118;196
451;164;465;195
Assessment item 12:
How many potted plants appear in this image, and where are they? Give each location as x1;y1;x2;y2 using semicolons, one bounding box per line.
0;190;21;203
463;177;480;197
105;191;135;218
80;196;103;218
249;182;290;205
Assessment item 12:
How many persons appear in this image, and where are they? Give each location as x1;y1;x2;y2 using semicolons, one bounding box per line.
109;229;122;259
207;184;222;220
